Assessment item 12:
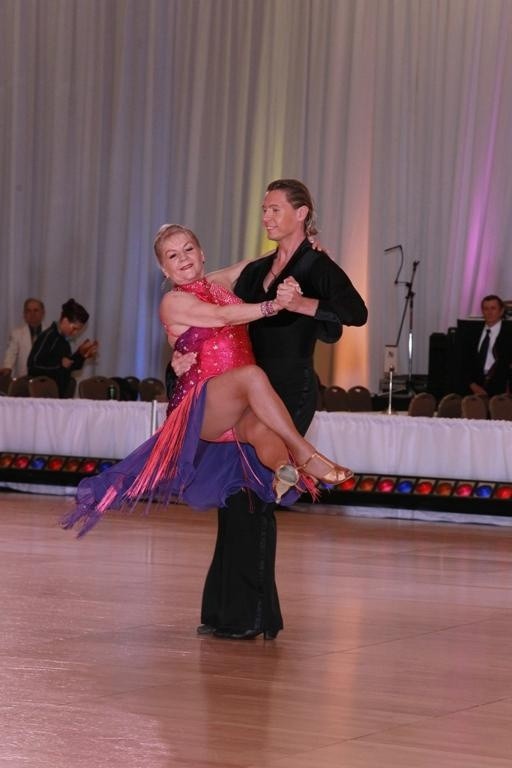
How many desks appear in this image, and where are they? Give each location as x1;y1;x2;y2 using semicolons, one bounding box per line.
0;398;168;484
295;410;512;527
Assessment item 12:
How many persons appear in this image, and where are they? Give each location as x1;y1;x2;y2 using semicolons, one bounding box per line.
170;179;368;644
452;295;512;397
27;298;98;397
2;298;50;380
58;224;355;540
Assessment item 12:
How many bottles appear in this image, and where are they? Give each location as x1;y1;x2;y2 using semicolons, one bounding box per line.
106;384;117;400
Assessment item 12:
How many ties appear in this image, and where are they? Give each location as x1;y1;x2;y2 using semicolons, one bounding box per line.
479;330;490;376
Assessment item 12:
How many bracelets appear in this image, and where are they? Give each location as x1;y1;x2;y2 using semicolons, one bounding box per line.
267;301;279;318
255;303;259;316
261;301;267;316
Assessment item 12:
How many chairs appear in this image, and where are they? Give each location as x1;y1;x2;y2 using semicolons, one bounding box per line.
79;376;168;403
0;376;59;399
317;385;373;411
408;392;512;421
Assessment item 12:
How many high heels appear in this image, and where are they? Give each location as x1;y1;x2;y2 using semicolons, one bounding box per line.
214;625;280;640
272;464;301;505
197;624;217;634
292;452;354;485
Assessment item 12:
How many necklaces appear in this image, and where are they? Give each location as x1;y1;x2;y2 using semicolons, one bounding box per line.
268;269;278;291
